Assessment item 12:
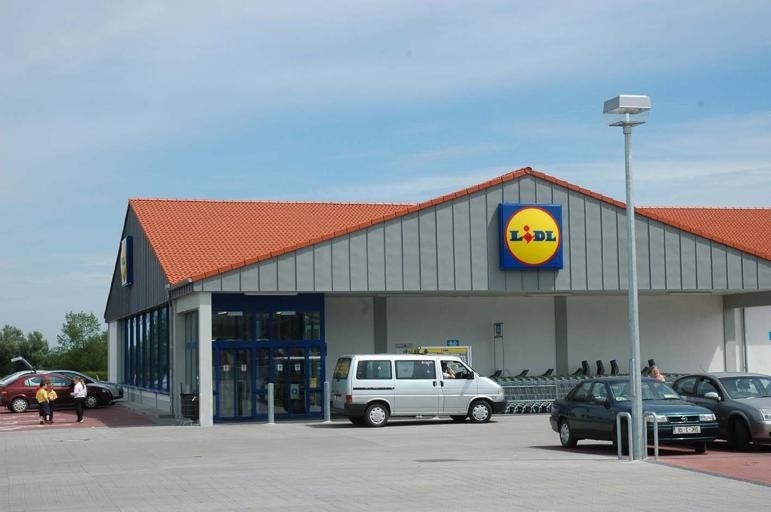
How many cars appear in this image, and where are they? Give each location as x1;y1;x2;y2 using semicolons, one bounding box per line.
550;373;721;454
0;356;124;399
0;374;112;413
672;372;771;450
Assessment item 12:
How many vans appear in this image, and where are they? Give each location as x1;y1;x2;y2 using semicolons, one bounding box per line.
331;354;508;428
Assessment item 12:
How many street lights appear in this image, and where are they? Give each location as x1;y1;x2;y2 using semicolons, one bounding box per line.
603;95;659;463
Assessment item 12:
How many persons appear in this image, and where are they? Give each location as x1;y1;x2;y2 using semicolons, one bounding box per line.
443;364;456;379
69;376;88;423
648;366;666;385
45;382;58;423
35;381;53;425
